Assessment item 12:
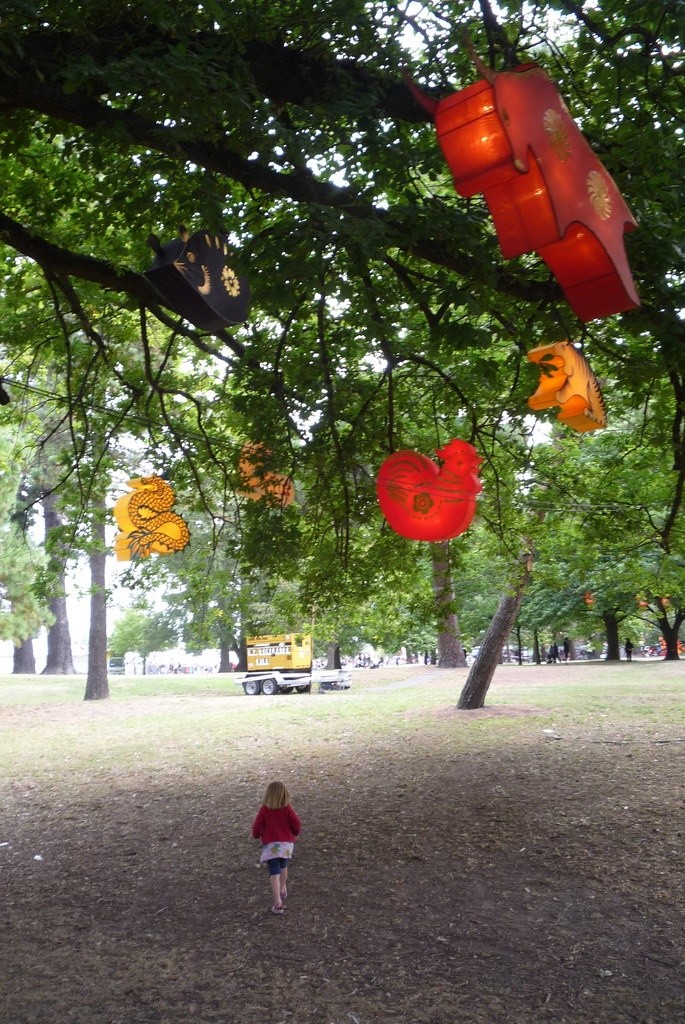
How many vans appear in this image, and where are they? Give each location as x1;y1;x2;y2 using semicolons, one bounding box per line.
106;656;125;675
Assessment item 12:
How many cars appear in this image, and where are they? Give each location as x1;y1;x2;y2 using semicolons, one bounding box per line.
545;647;587;662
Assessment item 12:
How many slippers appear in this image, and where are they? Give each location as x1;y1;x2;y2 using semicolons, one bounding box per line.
280;887;288;899
271;906;284;914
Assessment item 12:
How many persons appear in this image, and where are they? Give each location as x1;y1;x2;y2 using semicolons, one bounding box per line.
563;638;570;662
625;638;633;662
252;782;303;914
550;642;562;663
